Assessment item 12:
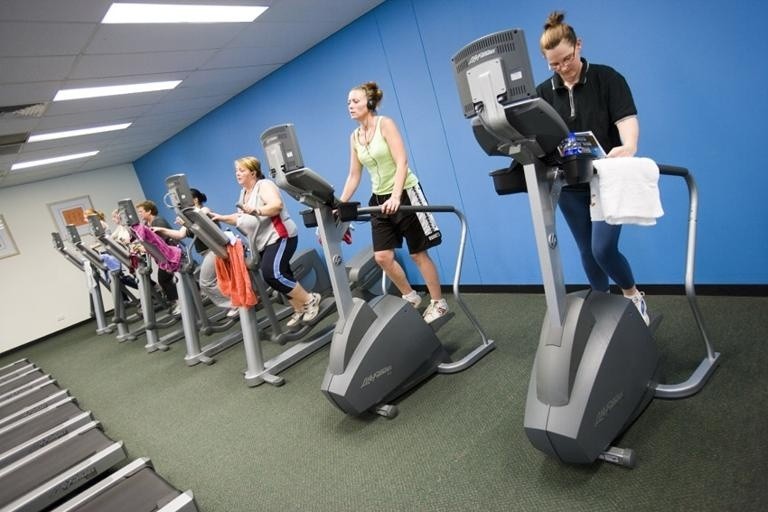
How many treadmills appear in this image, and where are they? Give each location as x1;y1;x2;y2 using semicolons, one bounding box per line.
1;367;50;398
0;359;34;377
0;397;94;467
0;420;129;512
52;458;197;512
1;379;69;429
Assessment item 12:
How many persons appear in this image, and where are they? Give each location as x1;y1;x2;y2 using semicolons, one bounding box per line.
105;207;163;301
147;186;240;321
81;208;139;304
525;10;651;330
135;200;184;318
333;80;450;326
205;157;321;326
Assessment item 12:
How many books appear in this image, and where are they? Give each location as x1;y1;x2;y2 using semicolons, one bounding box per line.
557;130;606;161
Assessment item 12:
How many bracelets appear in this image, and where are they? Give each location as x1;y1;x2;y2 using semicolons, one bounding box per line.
257;208;262;216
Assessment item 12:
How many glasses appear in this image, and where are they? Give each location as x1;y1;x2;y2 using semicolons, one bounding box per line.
547;43;575;70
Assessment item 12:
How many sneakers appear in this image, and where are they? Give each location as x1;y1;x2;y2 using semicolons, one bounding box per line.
626;291;650;326
423;297;449;324
286;311;303;327
403;294;421;309
302;292;321;322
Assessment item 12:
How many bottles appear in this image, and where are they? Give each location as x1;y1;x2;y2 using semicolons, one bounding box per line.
562;132;583;156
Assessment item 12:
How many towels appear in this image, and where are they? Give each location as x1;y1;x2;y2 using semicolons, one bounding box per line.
100;254;124;283
213;239;261;309
83;258;98;292
588;155;665;227
131;223;183;274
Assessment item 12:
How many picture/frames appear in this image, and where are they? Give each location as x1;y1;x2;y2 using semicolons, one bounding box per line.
46;195;96;242
0;213;21;261
